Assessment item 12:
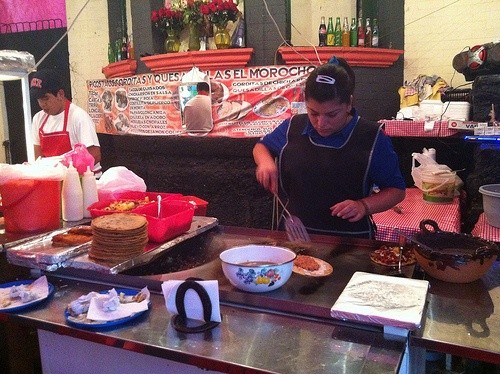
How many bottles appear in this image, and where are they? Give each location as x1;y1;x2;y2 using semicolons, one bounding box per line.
327;16;334;46
334;18;342;46
319;16;327;46
365;18;372;47
291;88;305;115
342;17;350;47
358;17;365;47
372;19;379;48
81;165;99;219
108;33;134;64
61;161;84;221
350;17;357;47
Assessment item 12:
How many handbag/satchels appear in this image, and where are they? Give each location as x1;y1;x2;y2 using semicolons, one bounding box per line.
411;148;463;189
470;73;500;123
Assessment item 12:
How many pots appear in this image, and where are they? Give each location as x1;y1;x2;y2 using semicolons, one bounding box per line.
412;219;499;285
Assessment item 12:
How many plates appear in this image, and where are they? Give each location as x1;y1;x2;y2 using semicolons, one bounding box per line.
100;87;130;134
210;81;290;119
0;278;53;312
65;289;151;328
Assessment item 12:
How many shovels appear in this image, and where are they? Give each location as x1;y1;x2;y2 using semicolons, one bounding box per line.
269;188;311;242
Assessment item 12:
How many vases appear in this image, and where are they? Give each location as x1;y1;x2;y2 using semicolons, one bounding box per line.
214;27;230;49
189;36;200;50
164;30;180;52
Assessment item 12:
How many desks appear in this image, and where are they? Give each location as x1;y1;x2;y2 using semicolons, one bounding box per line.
372;187;460;245
380;120;461;137
471;212;500;244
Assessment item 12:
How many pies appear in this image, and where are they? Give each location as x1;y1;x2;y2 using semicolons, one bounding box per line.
89;212;147;268
370;248;416;266
292;255;333;276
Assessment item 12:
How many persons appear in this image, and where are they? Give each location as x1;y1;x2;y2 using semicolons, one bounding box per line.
252;56;406;240
30;69;103;180
183;82;212;130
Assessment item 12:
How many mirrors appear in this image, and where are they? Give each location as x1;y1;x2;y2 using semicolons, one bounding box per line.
176;81;213;131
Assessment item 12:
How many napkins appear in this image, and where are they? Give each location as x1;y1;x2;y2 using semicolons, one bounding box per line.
68;287;152;323
162;279;223;324
9;283;38;303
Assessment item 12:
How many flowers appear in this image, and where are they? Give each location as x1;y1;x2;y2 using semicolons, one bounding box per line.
151;0;242;28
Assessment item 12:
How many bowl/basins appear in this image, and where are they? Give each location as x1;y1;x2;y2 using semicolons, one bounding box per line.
218;244;297;292
478;183;500;229
85;190;208;244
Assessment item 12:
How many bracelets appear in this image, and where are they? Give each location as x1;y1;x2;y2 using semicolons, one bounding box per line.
360;199;369;216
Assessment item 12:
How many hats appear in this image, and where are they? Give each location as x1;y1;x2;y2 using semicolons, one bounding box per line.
29;68;62;99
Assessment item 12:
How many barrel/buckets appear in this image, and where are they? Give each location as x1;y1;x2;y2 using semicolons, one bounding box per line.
419;171;457;205
0;177;63;236
